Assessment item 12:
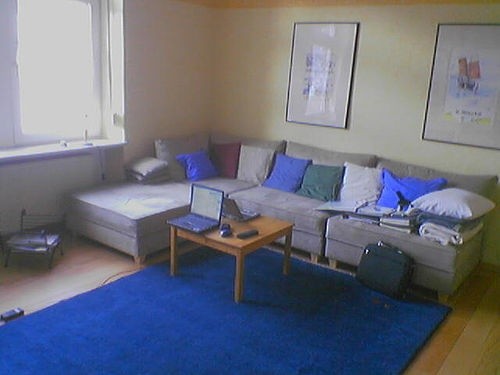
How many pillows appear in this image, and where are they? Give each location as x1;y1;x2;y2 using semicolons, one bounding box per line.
214;143;240;179
176;151;221;181
376;168;447;211
405;187;495;221
337;161;383;213
237;144;275;187
154;139;186;182
295;165;345;201
261;152;313;192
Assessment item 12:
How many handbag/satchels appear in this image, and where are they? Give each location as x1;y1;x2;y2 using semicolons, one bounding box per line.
356;240;416;302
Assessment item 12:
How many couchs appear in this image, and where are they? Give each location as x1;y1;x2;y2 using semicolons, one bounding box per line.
227;143;498;304
72;133;285;304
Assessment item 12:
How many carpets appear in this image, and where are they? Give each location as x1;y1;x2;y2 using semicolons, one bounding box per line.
1;246;452;375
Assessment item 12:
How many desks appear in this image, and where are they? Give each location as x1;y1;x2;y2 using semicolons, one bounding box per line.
167;215;294;303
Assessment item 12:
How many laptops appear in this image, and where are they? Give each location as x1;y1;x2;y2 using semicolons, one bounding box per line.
222;198;260;221
166;183;224;234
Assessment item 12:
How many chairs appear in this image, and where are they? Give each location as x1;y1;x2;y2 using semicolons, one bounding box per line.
4;208;66;269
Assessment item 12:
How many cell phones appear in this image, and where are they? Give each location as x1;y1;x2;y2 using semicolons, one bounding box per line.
1;308;24;320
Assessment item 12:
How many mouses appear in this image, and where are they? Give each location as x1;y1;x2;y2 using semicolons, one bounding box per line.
220;230;232;237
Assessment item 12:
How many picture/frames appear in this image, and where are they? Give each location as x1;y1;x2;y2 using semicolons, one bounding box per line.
285;21;360;129
420;22;500;151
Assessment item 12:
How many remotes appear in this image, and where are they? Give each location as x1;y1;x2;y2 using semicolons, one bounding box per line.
237;229;258;239
